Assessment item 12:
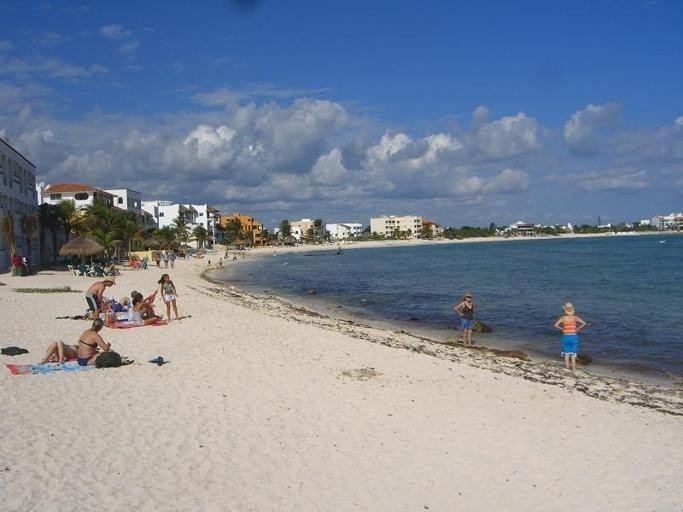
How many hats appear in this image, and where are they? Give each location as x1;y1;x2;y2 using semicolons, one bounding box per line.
104;276;115;285
463;293;472;297
562;302;575;316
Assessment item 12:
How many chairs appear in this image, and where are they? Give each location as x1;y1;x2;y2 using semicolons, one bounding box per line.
64;263;116;277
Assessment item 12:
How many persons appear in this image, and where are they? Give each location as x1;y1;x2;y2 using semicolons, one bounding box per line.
77;319;111;366
84;274;179;325
111;264;121;276
336;242;342;255
9;252;30;273
37;340;78;365
454;293;475;348
553;302;585;372
169;254;176;268
162;251;169;268
154;252;161;269
